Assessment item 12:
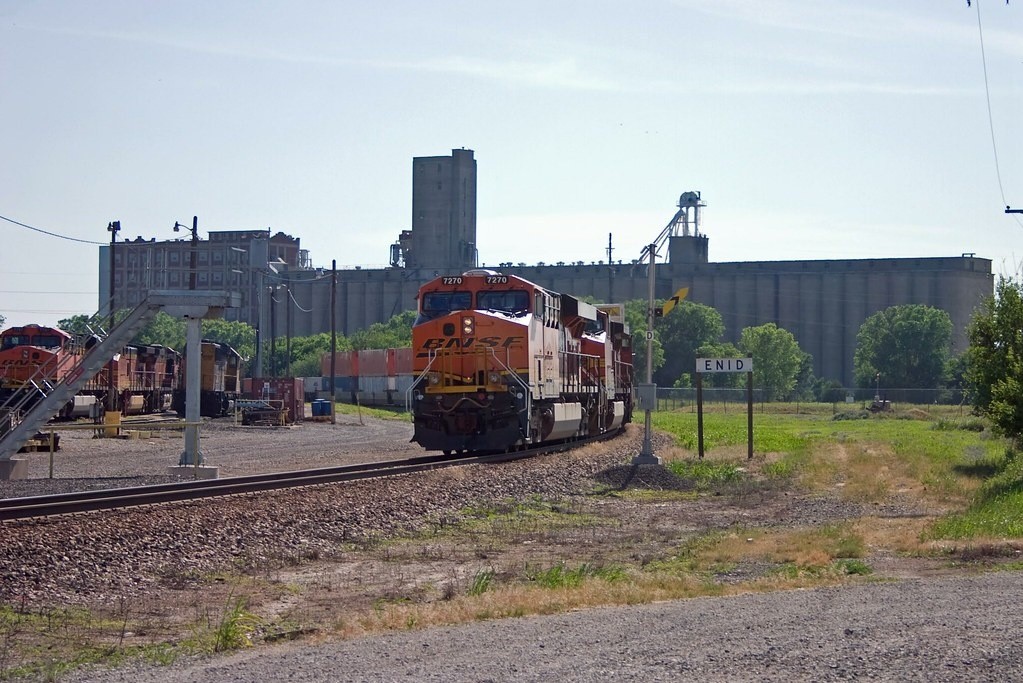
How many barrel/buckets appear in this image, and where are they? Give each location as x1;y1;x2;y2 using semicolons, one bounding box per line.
321;401;331;415
311;402;322;416
315;398;326;406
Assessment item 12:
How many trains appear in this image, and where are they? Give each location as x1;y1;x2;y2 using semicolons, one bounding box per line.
404;268;635;456
0;324;182;420
174;339;243;417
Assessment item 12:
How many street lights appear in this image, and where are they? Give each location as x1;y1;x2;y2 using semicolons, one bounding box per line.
173;216;198;289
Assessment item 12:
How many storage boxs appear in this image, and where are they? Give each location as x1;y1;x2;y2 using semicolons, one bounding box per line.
251;348;413;422
